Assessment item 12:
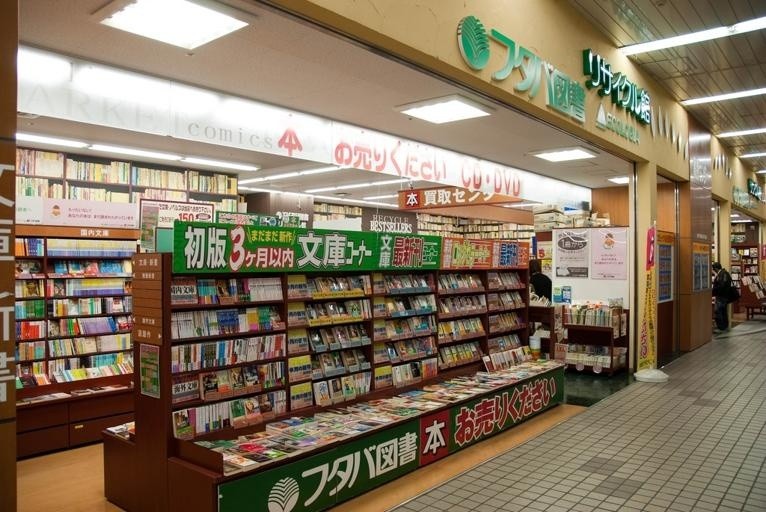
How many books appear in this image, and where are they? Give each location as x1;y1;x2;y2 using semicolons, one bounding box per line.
418;213;535;242
711;222;766;308
169;271;562;476
564;343;626;368
16;149;237;214
13;237;134;404
557;303;622;327
107;421;136;442
311;203;363;222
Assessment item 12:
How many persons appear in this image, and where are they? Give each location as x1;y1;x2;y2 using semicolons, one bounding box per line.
711;261;732;335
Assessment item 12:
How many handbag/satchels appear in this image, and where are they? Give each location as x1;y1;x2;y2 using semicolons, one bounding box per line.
726;286;740;304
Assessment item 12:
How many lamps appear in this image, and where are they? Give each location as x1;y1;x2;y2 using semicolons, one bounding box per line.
528;145;600;163
392;93;494;126
16;128;89;150
604;16;766;178
92;0;256;54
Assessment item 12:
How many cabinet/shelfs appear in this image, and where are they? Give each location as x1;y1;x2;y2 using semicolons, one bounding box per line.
560;306;630;378
18;139;565;511
712;242;766;320
